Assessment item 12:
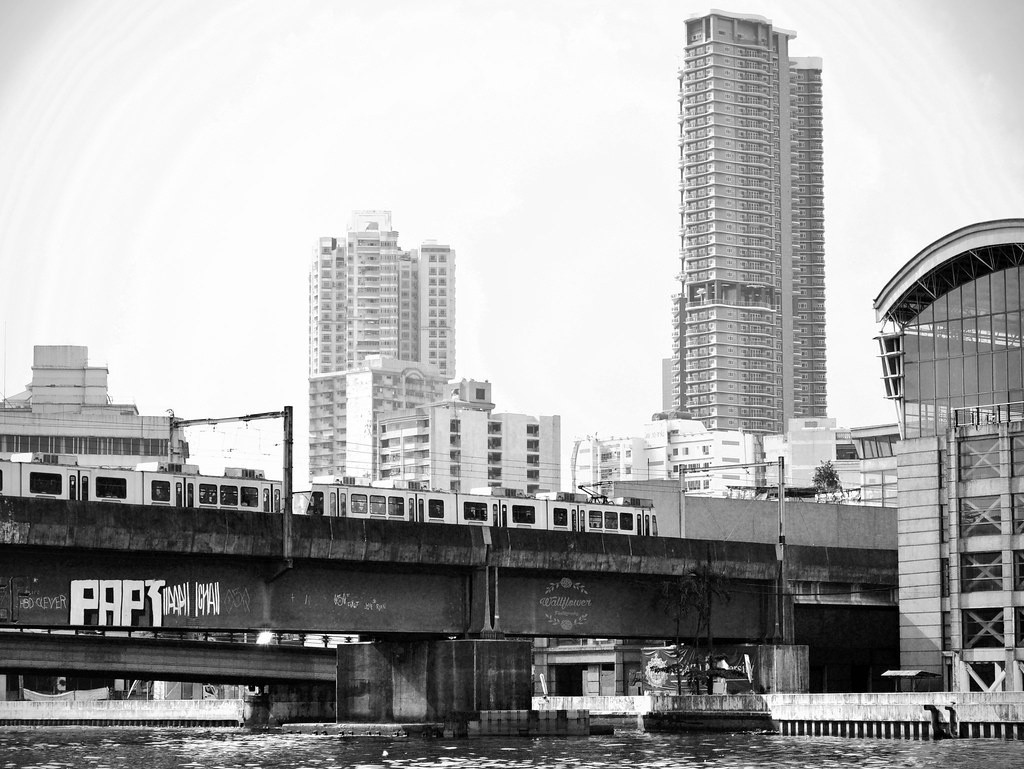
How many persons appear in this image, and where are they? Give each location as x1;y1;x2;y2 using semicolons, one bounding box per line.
351;503;366;512
220;490;234;505
263;489;269;511
605;516;618;529
589;522;602;528
430;504;440;517
199;484;217;504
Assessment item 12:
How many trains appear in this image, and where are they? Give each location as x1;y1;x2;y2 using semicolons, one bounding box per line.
0;453;659;539
